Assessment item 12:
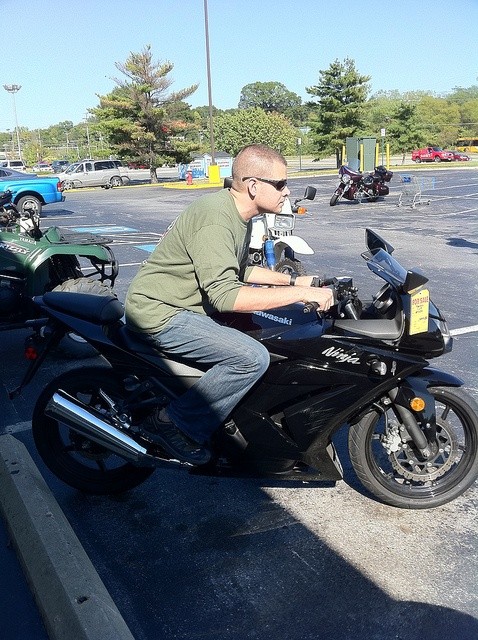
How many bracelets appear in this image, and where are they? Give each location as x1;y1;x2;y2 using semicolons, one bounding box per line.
290;272;301;286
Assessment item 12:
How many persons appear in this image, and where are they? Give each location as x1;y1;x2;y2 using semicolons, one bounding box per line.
124;143;335;466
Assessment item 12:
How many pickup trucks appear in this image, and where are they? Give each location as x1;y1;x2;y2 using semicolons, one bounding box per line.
411;147;454;163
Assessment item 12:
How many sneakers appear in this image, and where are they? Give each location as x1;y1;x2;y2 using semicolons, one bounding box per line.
141;405;210;464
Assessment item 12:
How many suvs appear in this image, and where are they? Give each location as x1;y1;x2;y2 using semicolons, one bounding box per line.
0;192;119;328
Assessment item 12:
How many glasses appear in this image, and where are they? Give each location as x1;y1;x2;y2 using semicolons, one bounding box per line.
243;175;286;190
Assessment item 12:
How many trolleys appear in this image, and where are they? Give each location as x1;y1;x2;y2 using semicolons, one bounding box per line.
396;172;435;209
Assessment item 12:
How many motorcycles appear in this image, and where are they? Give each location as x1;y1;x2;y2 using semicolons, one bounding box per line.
23;229;476;511
245;189;312;278
330;159;393;205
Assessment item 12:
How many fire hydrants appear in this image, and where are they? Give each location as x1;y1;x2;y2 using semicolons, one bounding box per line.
186;167;193;185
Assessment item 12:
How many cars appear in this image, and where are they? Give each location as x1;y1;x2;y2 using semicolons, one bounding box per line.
0;160;26;174
50;160;122;190
53;161;72;172
0;168;64;217
33;164;54;173
447;150;470;161
114;160;130;186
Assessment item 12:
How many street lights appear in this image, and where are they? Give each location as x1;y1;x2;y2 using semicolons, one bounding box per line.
3;83;22;160
